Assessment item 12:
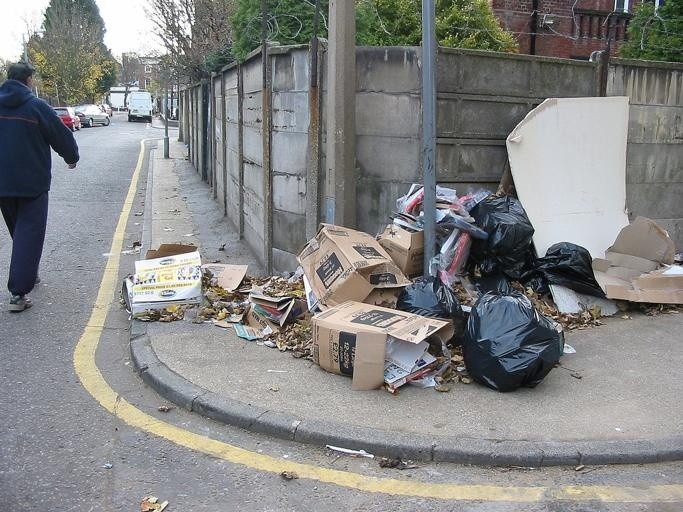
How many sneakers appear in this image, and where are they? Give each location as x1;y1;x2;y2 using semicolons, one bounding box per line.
8;297;33;311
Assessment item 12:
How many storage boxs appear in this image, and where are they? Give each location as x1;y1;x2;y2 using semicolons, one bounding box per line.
296;216;456;394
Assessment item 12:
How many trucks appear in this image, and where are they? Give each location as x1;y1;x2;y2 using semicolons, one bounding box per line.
128;91;152;122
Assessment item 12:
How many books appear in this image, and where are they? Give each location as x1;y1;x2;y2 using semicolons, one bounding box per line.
392;179;492;279
384;336;439;394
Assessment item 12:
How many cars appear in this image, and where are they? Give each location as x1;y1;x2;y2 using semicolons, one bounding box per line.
51;103;113;132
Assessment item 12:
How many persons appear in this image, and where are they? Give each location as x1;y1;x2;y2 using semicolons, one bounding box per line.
0;62;79;313
